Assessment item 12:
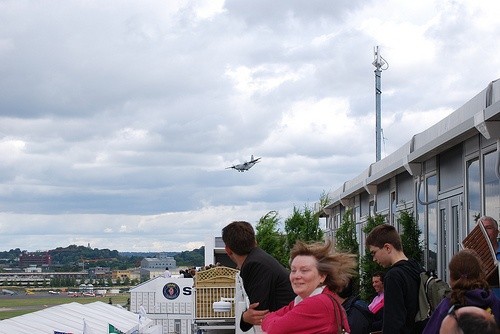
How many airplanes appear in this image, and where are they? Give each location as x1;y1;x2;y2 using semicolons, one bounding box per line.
225;155;262;172
473;213;481;223
25;289;35;296
2;289;14;296
48;290;61;296
67;292;97;297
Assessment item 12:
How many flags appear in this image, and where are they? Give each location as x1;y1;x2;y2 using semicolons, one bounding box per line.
139;304;149;326
54;331;73;334
109;324;126;334
83;321;91;334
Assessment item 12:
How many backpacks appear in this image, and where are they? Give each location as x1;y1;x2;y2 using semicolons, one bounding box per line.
398;259;454;326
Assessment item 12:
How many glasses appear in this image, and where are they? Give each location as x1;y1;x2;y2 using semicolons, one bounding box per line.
448;304;467;334
370;247;384;257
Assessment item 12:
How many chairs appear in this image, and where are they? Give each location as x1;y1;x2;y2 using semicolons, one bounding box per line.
459;220;500;287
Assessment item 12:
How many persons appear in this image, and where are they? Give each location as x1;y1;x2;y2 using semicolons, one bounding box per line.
439;304;500;334
222;221;299;332
421;248;500;334
261;238;358;334
367;224;426;334
163;267;171;278
179;263;239;279
331;275;370;334
367;270;385;334
476;216;500;263
